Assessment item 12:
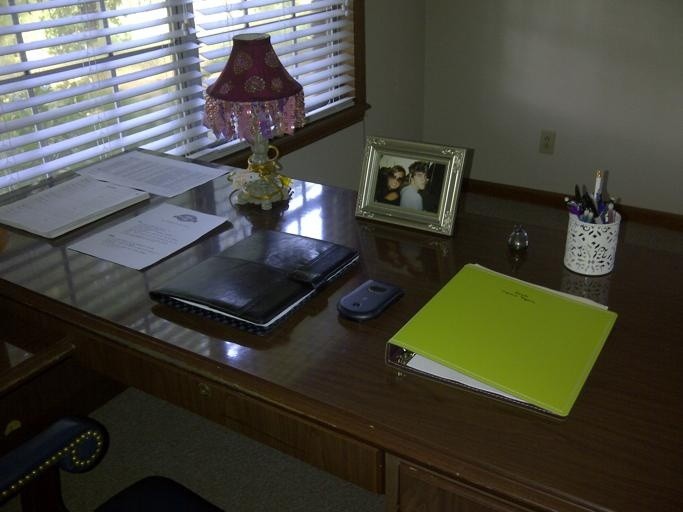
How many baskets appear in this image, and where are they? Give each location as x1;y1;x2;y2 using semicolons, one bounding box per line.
564;210;621;275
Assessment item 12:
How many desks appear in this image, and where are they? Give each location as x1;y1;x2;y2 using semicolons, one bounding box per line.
0;146;683;511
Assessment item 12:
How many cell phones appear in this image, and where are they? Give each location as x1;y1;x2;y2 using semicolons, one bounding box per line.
338;277;400;319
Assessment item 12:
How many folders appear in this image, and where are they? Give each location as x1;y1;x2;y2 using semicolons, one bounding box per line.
384;262;618;424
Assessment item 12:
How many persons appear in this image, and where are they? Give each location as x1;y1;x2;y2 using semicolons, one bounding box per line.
374;163;409;207
399;160;431;212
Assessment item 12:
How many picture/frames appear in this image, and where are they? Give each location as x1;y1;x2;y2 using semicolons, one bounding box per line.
353;137;468;237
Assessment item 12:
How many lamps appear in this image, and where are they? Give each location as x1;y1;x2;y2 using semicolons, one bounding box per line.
203;32;305;210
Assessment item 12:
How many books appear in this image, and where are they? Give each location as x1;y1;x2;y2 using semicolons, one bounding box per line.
145;227;360;339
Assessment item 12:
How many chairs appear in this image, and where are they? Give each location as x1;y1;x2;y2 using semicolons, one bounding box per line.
2;419;223;511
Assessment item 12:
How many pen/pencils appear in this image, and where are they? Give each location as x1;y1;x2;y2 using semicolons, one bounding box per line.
559;168;618;224
